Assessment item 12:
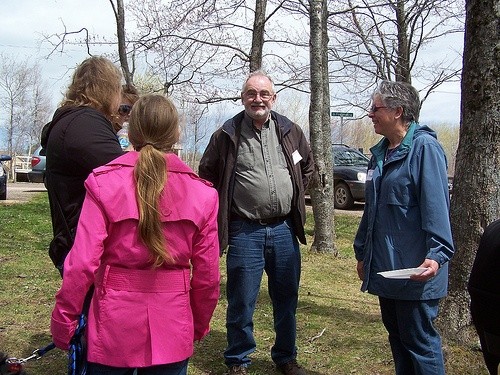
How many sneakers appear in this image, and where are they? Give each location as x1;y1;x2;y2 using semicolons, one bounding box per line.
276;360;305;375
230;366;248;375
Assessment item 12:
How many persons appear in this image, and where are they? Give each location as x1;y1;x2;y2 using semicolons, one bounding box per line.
353;80;455;375
50;95;219;375
466;219;500;375
41;56;129;375
112;84;140;133
198;68;315;375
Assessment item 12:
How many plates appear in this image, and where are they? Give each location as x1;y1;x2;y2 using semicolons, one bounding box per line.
377;268;428;278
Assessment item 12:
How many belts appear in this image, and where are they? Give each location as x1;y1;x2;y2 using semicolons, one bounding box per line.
242;214;290;226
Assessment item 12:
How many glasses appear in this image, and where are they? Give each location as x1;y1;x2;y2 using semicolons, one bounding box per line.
118;105;132;116
370;105;395;112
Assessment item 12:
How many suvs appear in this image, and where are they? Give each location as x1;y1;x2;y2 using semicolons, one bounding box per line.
331;141;371;210
30;144;48;179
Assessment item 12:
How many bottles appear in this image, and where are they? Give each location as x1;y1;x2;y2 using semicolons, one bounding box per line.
117;121;131;148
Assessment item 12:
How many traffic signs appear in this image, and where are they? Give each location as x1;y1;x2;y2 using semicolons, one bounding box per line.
332;112;353;116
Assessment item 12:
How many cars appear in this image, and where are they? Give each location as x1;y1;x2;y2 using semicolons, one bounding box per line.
0;155;11;204
